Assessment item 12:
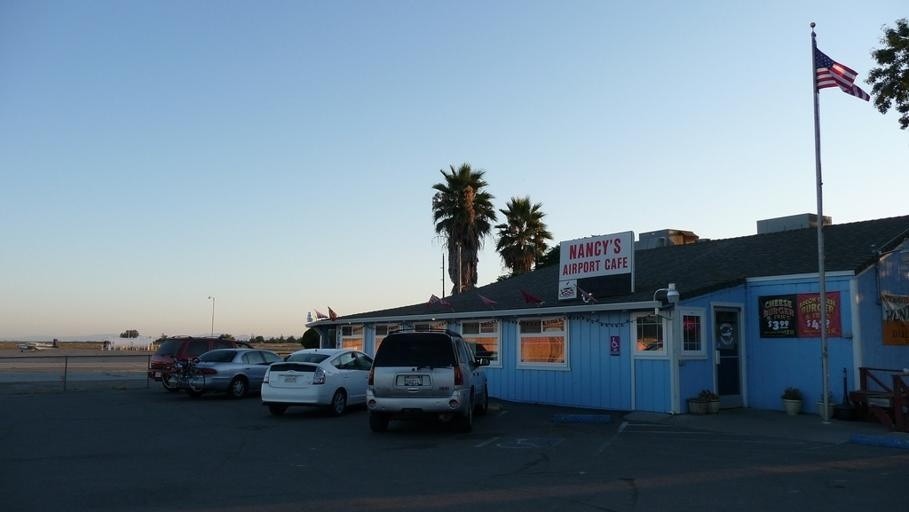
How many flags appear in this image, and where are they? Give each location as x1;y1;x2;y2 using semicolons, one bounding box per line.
328;307;337;321
521;291;541;303
428;295;451;308
314;310;328;318
479;295;497;306
814;47;871;103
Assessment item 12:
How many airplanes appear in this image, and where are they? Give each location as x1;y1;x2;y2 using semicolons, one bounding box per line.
17;337;59;352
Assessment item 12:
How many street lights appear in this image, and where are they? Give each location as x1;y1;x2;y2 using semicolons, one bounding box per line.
208;296;215;336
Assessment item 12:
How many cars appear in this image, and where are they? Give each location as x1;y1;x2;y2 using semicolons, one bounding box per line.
262;345;373;414
184;346;283;398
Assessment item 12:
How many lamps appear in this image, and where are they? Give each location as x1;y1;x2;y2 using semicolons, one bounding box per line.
653;282;680;321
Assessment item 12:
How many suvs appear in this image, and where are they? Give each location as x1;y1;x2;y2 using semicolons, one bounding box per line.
149;335;255;382
366;326;489;430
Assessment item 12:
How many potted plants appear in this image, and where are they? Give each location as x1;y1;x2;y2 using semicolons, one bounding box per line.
818;390;835;419
688;389;721;415
780;387;804;416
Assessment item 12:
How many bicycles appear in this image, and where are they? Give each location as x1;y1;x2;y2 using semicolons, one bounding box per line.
161;355;206;392
